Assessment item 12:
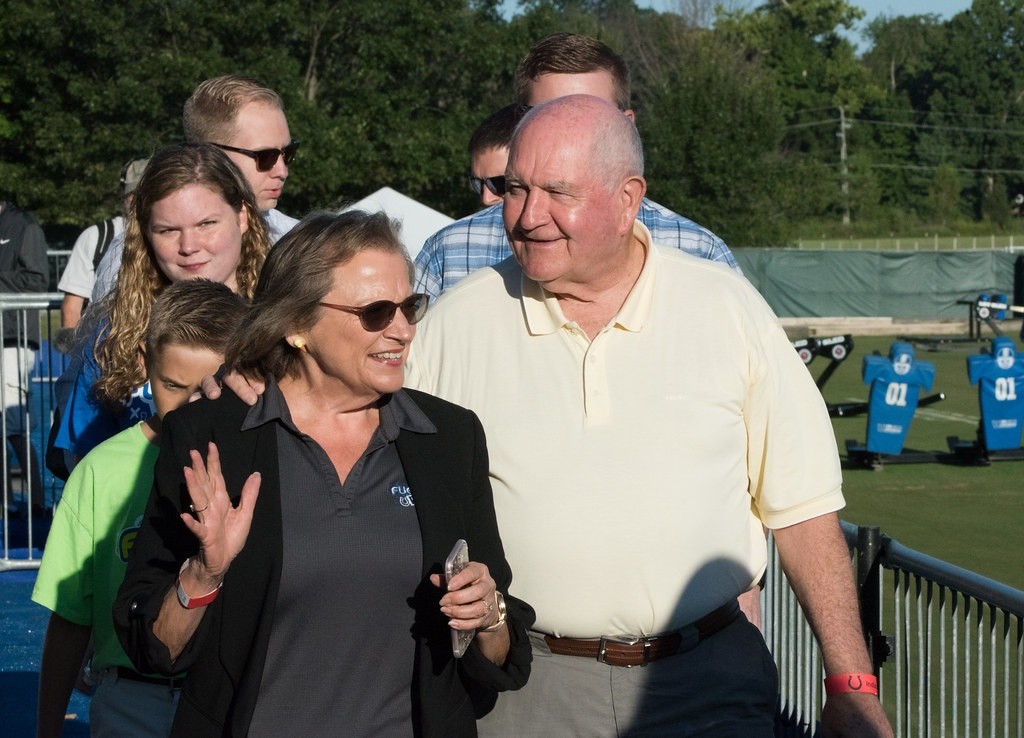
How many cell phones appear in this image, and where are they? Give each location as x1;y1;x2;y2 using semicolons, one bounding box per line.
445;538;475;658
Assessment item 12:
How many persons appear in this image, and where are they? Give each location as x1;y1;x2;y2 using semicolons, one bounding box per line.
30;33;892;738
0;197;51;521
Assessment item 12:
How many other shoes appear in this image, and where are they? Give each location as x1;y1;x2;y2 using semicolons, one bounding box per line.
31;500;44;518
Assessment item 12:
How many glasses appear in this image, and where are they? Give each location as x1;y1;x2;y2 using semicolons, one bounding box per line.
209;143;300;172
316;293;429;332
469;177;505;199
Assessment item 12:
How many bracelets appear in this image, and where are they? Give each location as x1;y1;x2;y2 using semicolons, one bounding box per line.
176;554;225;610
824;674;878;697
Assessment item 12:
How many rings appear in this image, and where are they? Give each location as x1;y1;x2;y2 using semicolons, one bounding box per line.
483;600;493;616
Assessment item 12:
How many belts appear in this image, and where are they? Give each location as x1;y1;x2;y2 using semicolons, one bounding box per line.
546;598;741;666
103;667;176;685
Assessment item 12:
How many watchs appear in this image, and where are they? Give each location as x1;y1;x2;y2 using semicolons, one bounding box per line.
479;588;507;633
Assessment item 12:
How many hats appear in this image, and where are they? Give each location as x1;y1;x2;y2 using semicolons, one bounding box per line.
125;159;150;195
